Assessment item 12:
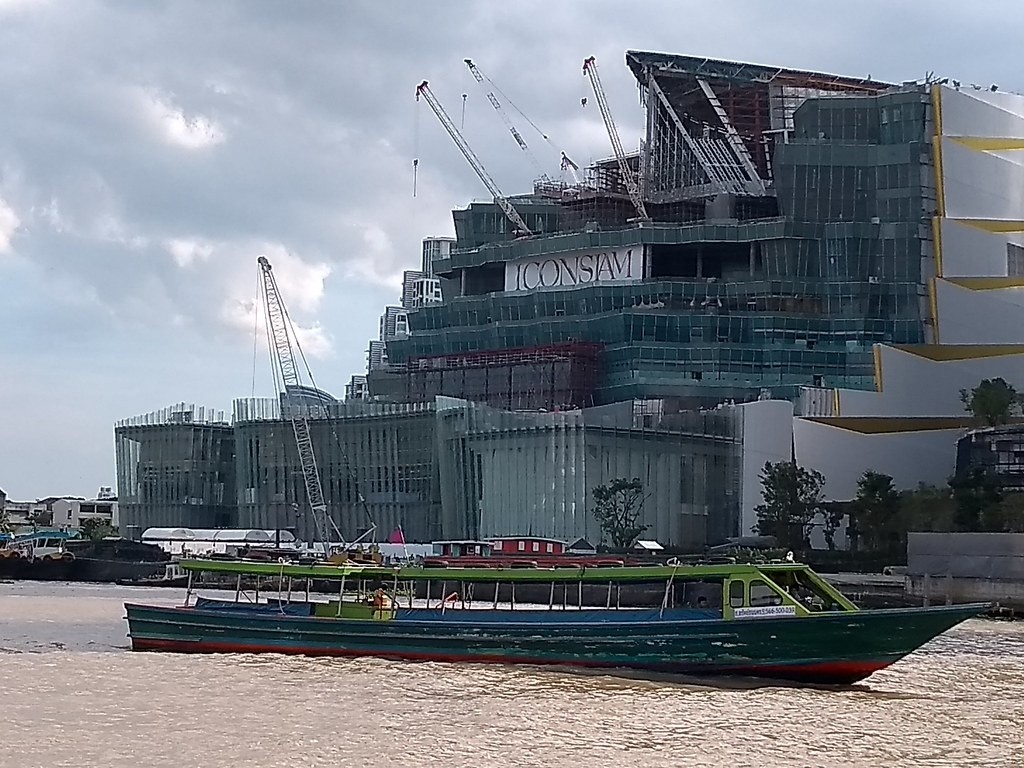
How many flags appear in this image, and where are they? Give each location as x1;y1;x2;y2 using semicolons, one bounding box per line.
390;526;404;543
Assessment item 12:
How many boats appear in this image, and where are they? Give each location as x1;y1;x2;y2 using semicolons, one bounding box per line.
121;555;993;688
